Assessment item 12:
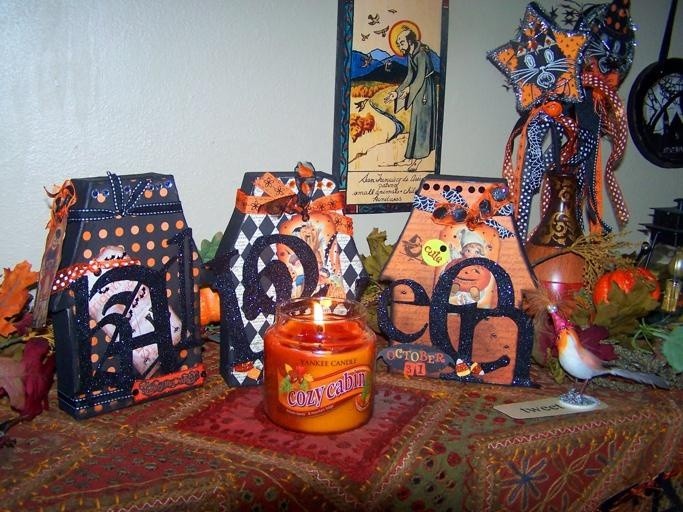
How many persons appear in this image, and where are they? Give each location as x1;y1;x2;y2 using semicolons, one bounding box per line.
384;25;441;166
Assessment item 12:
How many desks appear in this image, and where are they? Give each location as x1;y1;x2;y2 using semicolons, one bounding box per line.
2;340;682;512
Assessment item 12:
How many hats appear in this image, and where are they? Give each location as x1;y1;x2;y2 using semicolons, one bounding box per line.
459;228;485;248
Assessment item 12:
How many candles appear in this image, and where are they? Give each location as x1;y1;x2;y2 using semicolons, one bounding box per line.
264;298;377;435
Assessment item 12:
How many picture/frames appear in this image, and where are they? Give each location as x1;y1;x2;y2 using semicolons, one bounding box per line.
332;0;449;214
